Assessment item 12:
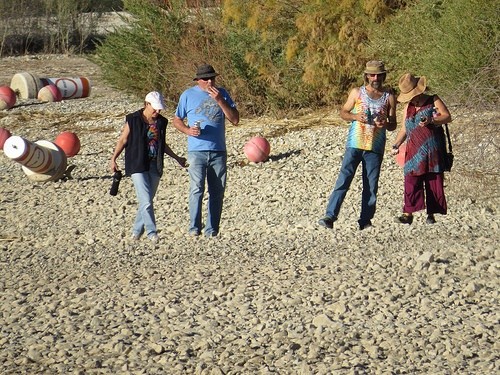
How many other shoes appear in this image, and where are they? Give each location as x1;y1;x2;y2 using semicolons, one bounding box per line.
189;230;199;236
131;233;139;240
208;230;217;236
148;232;159;241
318;217;333;229
358;218;371;226
399;214;413;223
427;215;435;223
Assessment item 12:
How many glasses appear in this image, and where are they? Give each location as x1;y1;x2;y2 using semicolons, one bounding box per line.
202;76;216;81
370;74;382;77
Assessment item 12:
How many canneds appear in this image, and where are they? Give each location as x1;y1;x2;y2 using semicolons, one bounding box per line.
194;121;200;135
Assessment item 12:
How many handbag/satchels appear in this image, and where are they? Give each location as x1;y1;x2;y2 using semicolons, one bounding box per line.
443;153;454;172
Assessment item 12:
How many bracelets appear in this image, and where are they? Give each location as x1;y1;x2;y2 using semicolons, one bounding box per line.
393;145;399;149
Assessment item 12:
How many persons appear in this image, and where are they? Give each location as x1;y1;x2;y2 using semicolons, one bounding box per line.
391;73;452;224
173;63;239;237
110;91;190;241
318;60;397;230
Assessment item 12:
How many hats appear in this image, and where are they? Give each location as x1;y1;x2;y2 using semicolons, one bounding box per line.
193;64;220;81
396;73;426;103
363;60;388;74
145;91;166;112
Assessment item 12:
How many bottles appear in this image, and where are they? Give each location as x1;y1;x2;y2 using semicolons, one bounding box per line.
110;170;122;195
365;108;372;124
194;120;200;136
420;115;425;122
